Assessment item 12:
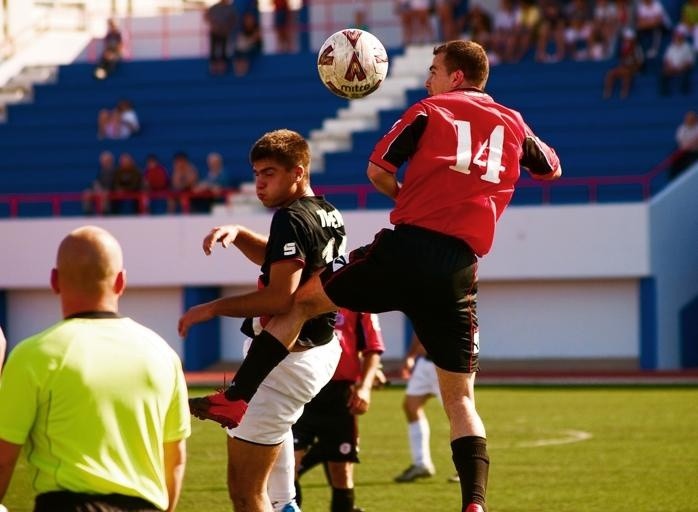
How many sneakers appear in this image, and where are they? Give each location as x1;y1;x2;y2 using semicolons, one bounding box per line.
187;387;249;429
449;472;461;482
394;460;438;483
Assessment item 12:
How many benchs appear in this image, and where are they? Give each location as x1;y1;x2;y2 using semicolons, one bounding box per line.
484;56;697;207
1;42;437;216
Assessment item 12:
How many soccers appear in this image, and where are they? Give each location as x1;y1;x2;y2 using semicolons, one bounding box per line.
318;28;388;99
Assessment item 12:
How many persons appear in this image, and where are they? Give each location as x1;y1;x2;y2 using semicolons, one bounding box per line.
289;306;387;512
271;0;293;55
96;97;141;142
187;39;563;512
76;148;228;214
232;13;264;77
202;0;238;76
344;6;369;32
669;110;698;178
393;328;443;483
176;126;344;512
94;15;125;79
0;223;193;512
